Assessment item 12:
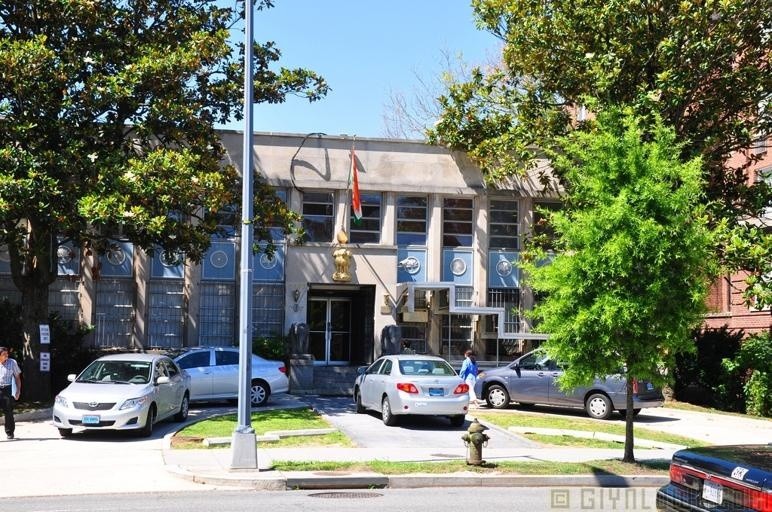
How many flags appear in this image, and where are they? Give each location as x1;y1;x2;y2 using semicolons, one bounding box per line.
351;149;364;220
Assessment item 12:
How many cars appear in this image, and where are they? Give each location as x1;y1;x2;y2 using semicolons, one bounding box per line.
173;346;289;407
474;347;665;419
53;354;190;439
353;354;469;427
656;443;772;512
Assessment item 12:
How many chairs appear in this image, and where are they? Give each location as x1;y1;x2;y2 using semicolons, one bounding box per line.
432;367;445;374
403;366;413;372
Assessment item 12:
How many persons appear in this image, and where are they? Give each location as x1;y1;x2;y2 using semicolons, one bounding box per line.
0;344;21;440
459;349;480;409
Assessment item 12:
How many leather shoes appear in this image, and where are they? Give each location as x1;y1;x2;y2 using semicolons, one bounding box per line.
7;432;14;439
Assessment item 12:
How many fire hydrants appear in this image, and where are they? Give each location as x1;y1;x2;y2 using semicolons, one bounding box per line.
461;418;490;464
